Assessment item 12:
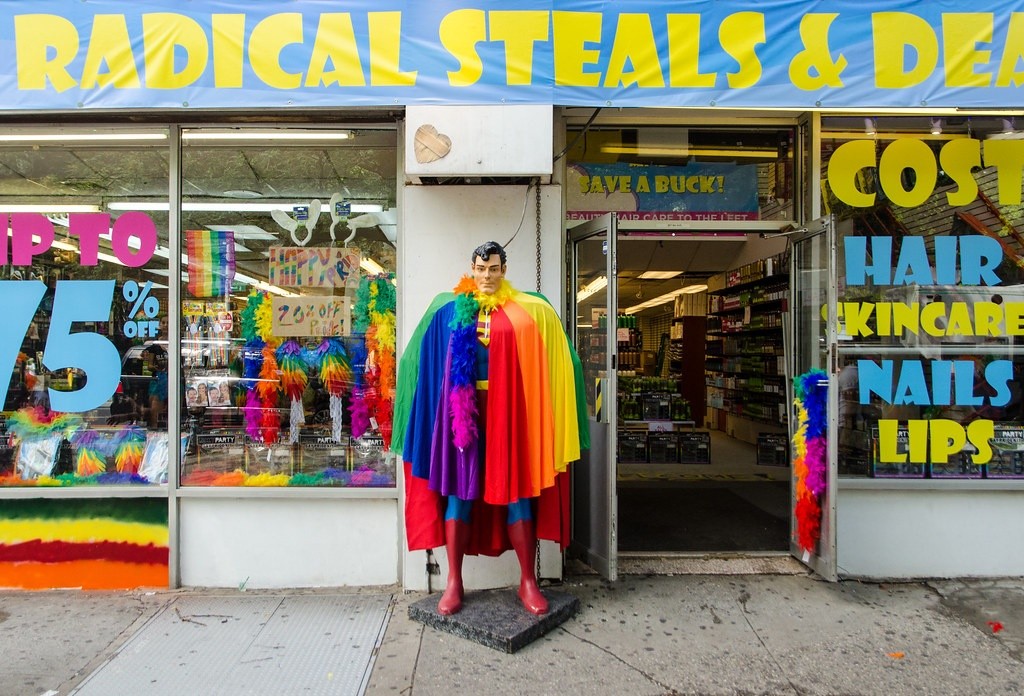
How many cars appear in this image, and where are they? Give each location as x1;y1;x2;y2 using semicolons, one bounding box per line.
836;360;921;460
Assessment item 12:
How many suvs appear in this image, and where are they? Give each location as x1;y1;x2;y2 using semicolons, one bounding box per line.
109;337;353;437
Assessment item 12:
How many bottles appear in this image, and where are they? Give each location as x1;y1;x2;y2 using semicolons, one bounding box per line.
598;313;637;365
723;319;728;333
708;295;723;313
727;256;788;375
707;316;721;334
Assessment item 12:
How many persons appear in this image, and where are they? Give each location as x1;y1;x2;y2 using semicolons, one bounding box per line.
25;358;35;377
209;381;230;406
108;345;184;430
392;241;592;614
188;383;208;406
148;315;168;377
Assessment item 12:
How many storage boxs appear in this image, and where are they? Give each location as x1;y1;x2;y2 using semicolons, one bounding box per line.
868;422;1024;480
707;272;727;292
643;350;658;375
756;431;789;467
617;430;712;465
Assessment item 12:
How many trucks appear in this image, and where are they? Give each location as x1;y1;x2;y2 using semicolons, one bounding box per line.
884;284;1024;424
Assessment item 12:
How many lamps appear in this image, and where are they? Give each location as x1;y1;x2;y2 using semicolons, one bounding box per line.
102;197;392;214
864;118;876;137
577;272;609;305
46;213;301;298
0;128;171;140
1;196;105;214
929;117;943;135
1000;118;1016;136
181;129;361;141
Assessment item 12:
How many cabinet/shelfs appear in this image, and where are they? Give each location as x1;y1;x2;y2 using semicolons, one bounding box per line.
836;268;922;452
704;273;791;446
669;316;707;428
